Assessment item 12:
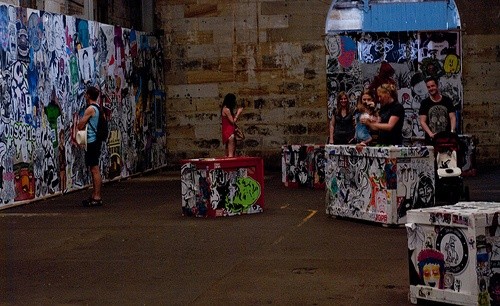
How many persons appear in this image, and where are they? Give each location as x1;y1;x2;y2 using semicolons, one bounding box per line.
352;90;377;145
360;84;405;147
329;91;353;145
221;93;243;157
77;87;108;207
419;77;456;144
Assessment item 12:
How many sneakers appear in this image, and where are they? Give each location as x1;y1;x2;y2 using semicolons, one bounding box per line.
82;195;104;209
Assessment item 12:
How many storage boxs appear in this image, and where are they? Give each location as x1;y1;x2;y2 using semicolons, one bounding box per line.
280;143;327;190
324;144;436;228
179;157;267;218
405;201;500;306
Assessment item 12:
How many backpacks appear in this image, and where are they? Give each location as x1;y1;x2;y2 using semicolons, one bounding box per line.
85;103;112;143
